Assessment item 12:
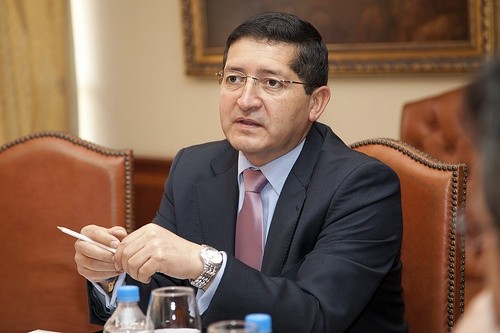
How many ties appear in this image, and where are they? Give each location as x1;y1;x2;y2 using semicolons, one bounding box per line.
233;169;267;272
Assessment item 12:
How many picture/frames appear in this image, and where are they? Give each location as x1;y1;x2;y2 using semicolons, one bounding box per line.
180;0;500;78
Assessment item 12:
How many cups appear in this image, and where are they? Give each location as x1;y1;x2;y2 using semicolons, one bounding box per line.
206;320;259;333
146;286;201;333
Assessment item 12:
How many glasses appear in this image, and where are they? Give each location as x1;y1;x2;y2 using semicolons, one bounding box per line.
214;70;322;95
453;209;499;251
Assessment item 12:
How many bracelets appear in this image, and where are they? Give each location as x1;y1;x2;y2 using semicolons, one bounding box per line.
103;273;119;294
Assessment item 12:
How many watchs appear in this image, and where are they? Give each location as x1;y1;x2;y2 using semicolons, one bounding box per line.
188;242;224;289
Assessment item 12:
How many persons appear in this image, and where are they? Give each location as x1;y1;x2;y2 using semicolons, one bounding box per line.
74;8;411;332
455;51;500;333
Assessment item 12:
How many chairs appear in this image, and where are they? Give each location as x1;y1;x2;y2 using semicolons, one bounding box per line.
0;129;135;333
399;87;484;308
348;136;468;333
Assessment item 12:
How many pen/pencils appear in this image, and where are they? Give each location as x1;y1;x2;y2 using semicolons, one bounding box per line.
55;225;116;254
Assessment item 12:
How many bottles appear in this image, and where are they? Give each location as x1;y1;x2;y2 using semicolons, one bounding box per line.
244;313;273;333
102;285;153;333
